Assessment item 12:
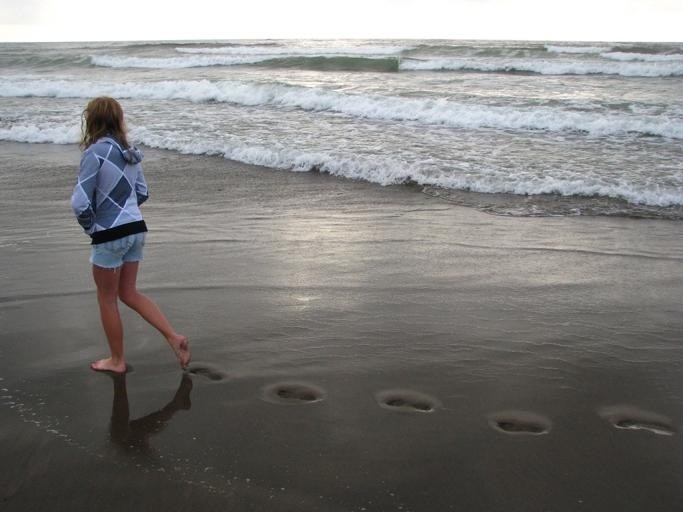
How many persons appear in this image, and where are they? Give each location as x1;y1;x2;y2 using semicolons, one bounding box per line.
104;372;193;462
68;96;193;375
